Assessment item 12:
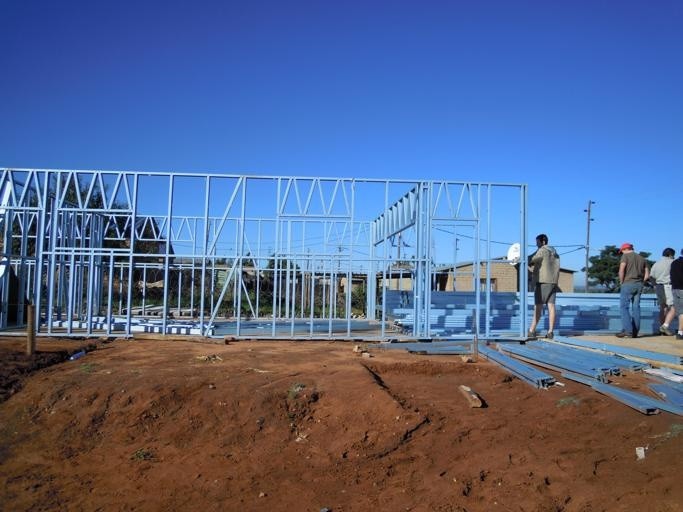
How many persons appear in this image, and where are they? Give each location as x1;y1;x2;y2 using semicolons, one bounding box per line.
512;232;560;341
614;243;649;339
647;246;677;336
669;248;683;341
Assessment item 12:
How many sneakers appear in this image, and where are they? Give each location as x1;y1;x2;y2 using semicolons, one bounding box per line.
616;329;639;338
655;324;683;340
528;329;553;339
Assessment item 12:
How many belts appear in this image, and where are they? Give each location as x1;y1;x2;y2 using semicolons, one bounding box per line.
655;283;673;286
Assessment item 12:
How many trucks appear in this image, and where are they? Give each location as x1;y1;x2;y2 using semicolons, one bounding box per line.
216;266;268;291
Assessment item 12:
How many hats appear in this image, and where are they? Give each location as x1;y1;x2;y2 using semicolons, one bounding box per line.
618;243;633;254
